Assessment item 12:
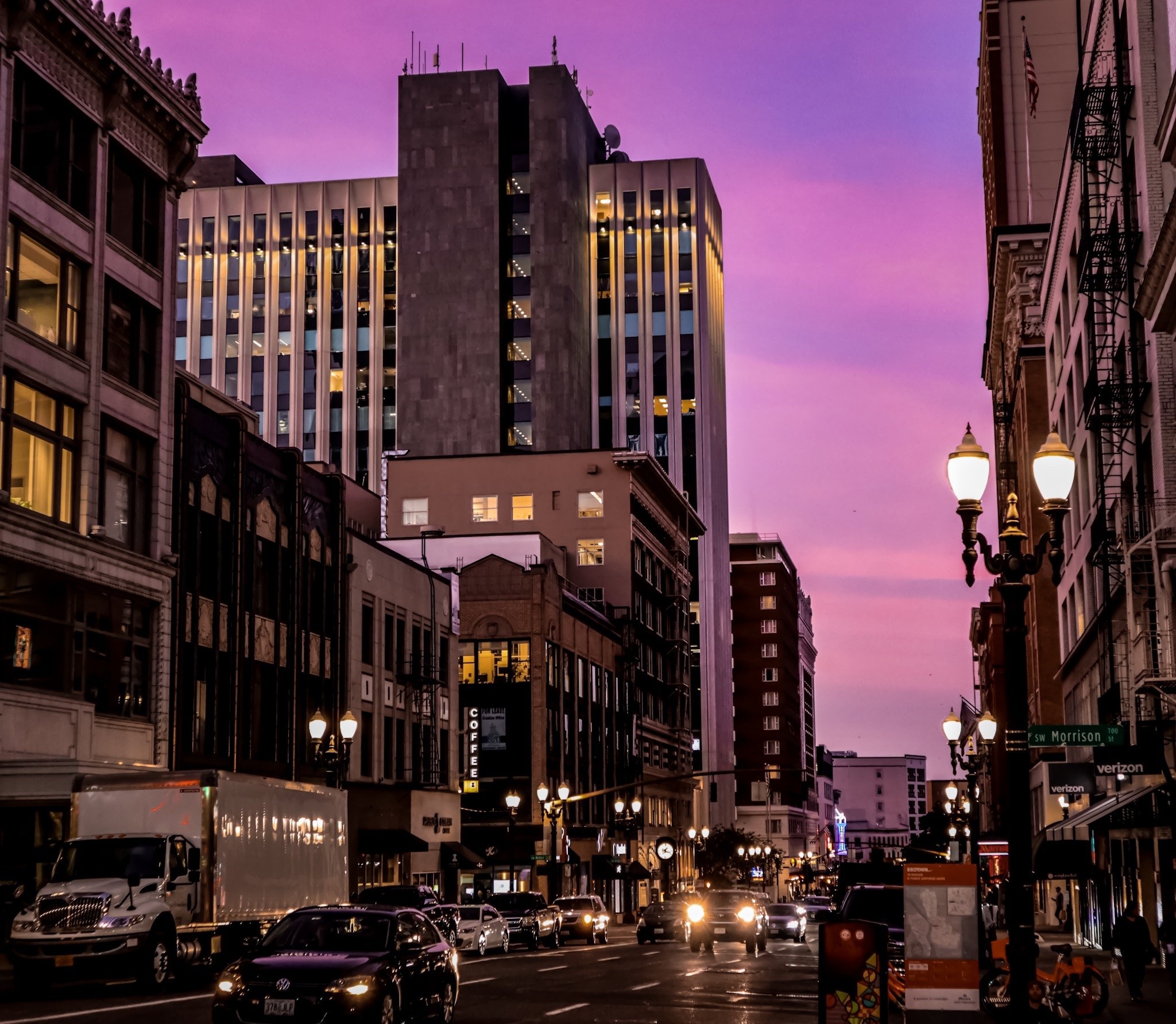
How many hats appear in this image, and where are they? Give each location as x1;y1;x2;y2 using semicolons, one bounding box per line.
1055;887;1061;890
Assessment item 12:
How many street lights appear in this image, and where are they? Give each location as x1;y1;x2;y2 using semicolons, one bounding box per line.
688;824;710;879
944;419;1076;1024
944;704;997;936
536;781;570;905
308;708;359;788
738;844;771;893
798;850;814;894
614;795;642;924
505;789;521;891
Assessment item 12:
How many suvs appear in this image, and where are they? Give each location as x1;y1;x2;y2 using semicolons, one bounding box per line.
836;883;905;975
551;893;609;946
687;889;768;953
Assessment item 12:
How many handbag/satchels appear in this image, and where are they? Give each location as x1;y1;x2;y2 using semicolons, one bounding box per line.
1058;910;1066;920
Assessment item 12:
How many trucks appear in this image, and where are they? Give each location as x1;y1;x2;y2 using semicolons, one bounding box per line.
3;768;350;996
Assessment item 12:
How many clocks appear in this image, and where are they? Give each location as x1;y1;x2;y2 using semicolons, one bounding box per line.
658;844;673;859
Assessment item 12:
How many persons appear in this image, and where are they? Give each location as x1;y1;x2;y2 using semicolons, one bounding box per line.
1110;900;1155;1004
125;847;177;877
1157;900;1176;997
980;895;990;921
1050;887;1064;928
795;886;822;896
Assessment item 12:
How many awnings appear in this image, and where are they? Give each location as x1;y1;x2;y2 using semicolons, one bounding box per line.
358;828;429;854
591;854;622;880
629;861;653;879
440;841;485;869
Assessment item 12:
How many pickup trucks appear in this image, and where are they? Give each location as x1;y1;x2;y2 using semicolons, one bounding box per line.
485;890;562;950
351;885;460;950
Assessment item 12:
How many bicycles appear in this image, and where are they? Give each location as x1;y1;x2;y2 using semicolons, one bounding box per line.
978;934;1110;1020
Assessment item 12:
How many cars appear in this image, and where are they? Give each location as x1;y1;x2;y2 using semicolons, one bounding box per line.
211;904;460;1024
766;902;808;943
448;904;510;958
635;901;688;944
802;894;833;919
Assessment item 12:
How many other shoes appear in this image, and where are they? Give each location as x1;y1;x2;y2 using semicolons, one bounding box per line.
1138;991;1143;999
1131;996;1137;1002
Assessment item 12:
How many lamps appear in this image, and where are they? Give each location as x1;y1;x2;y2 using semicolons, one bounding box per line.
1058;796;1069;820
